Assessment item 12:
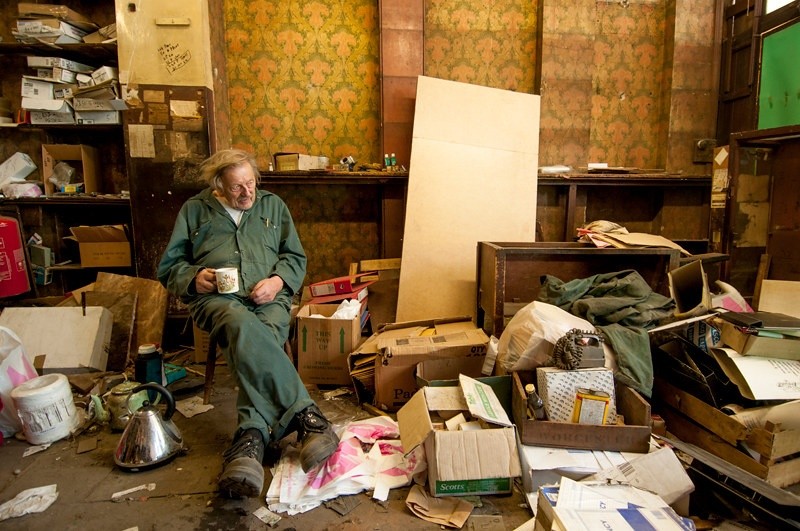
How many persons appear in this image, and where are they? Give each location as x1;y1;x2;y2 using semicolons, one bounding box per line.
156;149;339;502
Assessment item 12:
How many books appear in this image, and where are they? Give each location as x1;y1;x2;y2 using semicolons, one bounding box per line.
592;232;693;258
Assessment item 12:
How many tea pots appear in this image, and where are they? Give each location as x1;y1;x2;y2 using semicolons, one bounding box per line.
90;373;161;430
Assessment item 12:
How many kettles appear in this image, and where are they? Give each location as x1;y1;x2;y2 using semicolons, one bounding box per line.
112;380;187;472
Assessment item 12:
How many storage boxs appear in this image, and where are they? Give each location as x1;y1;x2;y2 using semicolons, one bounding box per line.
10;1;124;125
60;222;133;268
185;260;800;530
1;213;32;299
41;141;105;198
0;304;113;375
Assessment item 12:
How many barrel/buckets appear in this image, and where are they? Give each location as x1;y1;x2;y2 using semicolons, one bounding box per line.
11;373;78;445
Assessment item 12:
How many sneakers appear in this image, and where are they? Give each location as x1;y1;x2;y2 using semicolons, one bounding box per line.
217;428;265;498
294;404;341;473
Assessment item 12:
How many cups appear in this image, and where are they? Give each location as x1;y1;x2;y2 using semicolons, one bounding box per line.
210;267;239;294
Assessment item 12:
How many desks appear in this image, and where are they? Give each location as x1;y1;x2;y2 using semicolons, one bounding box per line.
247;163;713;237
475;240;682;340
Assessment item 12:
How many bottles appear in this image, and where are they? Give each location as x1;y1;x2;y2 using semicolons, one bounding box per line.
526;383;544;420
134;342;164;406
